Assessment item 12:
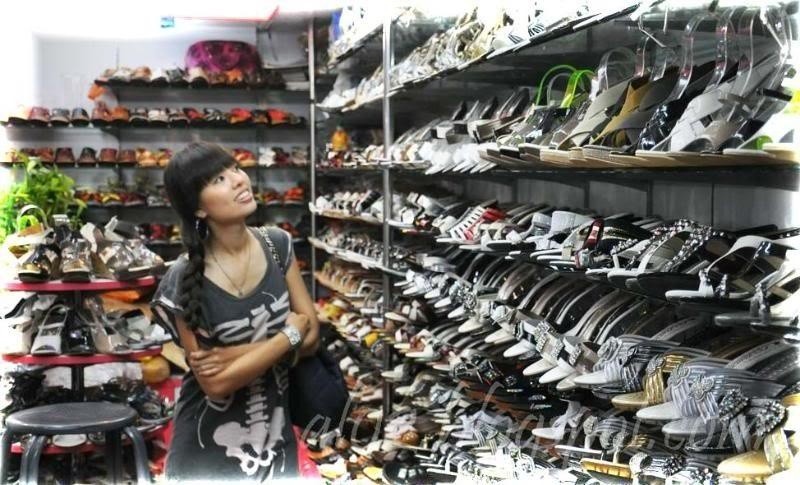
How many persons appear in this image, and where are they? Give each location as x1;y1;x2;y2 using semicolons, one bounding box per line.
149;141;322;481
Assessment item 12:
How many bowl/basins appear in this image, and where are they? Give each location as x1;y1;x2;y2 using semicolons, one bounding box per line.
207;234;251;298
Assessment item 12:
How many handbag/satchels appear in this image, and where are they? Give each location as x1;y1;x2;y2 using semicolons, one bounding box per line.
280;323;303;351
292;347;353;432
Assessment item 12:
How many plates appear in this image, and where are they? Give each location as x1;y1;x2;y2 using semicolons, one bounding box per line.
0;256;172;485
0;80;310;251
310;8;800;484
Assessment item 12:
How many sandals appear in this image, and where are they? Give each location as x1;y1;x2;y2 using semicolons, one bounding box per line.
5;295;173;355
309;191;797;484
312;6;797;176
17;227;166;282
0;64;308;241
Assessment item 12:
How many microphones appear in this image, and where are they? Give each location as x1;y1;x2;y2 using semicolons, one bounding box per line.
0;400;151;485
